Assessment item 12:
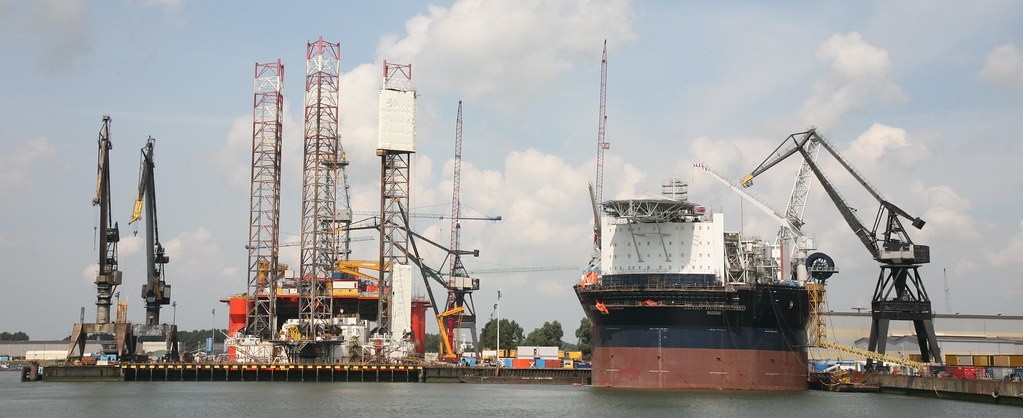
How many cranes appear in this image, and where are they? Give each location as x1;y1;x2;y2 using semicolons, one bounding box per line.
743;126;944;371
66;115;180;360
217;37;502;367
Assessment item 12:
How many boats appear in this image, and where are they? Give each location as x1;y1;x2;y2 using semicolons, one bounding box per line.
572;39;821;391
